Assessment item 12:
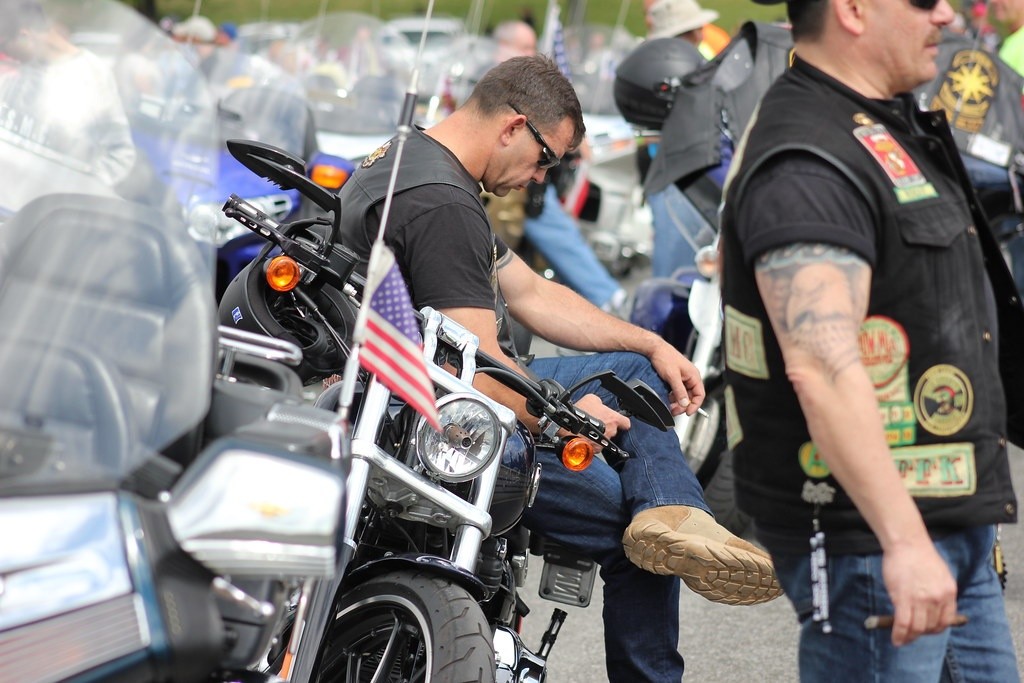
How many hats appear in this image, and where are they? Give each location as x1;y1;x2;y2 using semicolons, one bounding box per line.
644;2;720;38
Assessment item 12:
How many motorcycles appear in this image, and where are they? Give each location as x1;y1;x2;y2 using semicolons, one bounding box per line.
0;0;742;683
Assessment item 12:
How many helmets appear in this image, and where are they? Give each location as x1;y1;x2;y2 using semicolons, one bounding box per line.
209;215;360;375
609;35;699;123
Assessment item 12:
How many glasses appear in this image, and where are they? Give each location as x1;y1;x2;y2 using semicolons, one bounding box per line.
506;99;562;170
908;0;939;10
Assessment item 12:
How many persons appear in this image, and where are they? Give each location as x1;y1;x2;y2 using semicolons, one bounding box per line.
720;1;1024;683
0;0;721;321
318;53;787;682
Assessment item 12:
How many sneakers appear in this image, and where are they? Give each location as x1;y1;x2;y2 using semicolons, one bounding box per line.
621;501;787;608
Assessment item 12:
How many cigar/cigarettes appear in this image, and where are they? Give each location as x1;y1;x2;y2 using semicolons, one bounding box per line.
864;612;969;631
686;401;712;419
